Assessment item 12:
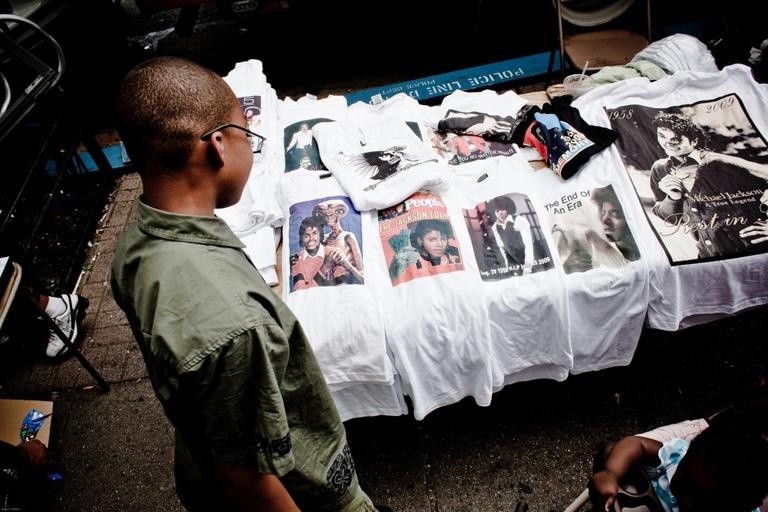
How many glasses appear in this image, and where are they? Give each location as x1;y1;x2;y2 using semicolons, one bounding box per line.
200;123;267;155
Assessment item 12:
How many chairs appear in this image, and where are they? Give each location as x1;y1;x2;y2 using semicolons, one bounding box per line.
547;2;650;91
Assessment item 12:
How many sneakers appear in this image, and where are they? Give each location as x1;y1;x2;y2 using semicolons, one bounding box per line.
45;294;90;364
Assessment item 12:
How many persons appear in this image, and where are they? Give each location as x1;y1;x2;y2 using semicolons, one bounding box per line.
0;440;60;512
110;55;378;512
292;216;340;291
285;123;313;169
649;114;768;259
587;414;768;512
492;201;546;277
290;199;364;284
27;288;90;360
562;189;639;274
413;219;460;270
